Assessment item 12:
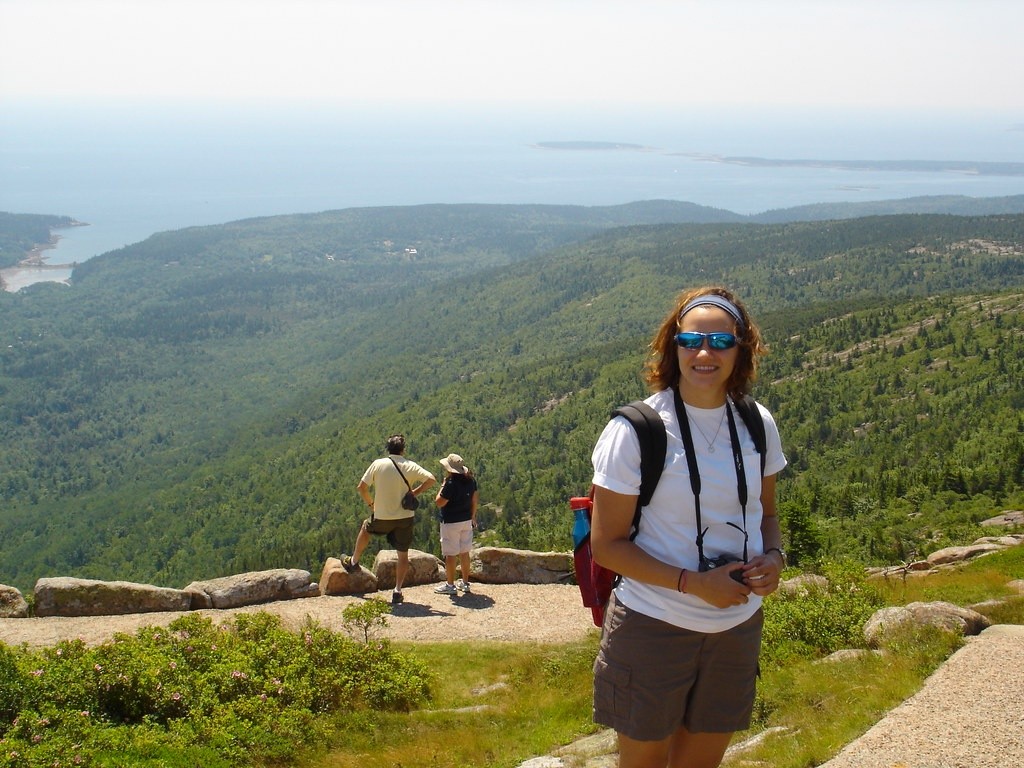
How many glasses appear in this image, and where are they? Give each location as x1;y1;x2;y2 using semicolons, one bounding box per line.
674;331;742;351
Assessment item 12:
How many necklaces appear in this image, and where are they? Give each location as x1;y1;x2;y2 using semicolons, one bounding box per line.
686;404;726;453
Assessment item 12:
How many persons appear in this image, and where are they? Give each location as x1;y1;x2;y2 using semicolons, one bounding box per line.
434;453;478;595
340;434;436;607
589;287;786;768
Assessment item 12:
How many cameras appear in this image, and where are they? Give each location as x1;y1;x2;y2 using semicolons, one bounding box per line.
699;554;748;586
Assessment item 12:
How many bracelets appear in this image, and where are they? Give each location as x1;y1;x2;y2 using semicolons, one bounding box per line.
368;502;374;507
441;485;445;487
677;569;688;594
764;548;787;570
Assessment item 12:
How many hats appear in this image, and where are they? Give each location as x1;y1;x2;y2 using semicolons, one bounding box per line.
439;453;468;474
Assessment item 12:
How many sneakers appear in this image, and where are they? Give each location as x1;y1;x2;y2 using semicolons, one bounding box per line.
340;554;361;573
392;589;403;604
434;581;458;595
456;581;470;591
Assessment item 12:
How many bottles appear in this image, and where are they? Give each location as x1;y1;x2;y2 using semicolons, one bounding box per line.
570;497;606;607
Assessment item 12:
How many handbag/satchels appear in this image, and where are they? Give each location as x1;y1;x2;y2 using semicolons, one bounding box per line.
402;494;419;510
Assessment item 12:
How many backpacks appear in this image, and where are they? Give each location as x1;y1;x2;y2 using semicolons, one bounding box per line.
572;395;767;627
